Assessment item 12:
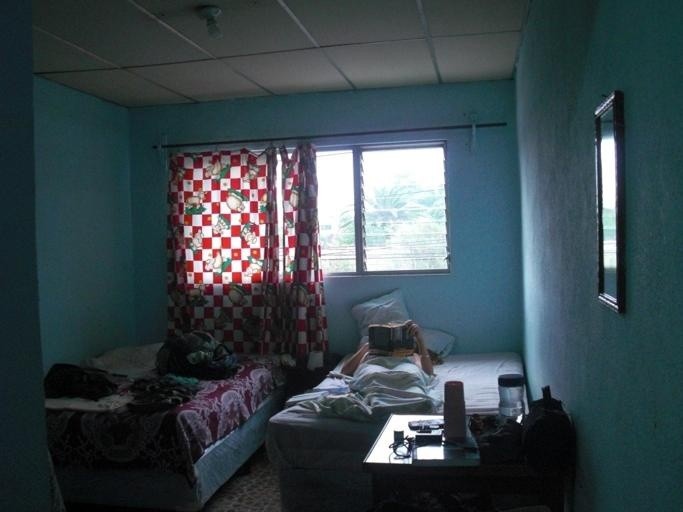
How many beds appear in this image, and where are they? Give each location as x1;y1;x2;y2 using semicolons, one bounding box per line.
44;343;284;512
265;350;531;512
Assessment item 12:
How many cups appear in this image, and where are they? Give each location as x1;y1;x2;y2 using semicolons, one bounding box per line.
443;380;468;444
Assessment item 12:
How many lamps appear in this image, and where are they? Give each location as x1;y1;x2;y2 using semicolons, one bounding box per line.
193;5;228;43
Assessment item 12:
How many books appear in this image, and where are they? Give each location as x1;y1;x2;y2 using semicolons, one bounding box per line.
368;323;417;357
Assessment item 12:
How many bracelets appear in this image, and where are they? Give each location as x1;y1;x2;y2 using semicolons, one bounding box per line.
419;353;430;359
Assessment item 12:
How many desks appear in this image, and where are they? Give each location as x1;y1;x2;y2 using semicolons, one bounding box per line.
361;412;578;511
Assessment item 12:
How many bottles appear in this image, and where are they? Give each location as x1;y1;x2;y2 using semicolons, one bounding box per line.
498;373;527;424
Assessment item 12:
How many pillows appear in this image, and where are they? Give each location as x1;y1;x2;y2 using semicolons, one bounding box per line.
418;323;456;361
350;288;412;341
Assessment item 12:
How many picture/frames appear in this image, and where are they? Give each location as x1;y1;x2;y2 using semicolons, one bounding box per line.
591;91;627;314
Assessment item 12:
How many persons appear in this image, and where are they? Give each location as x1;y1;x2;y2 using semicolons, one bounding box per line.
340;320;436;413
